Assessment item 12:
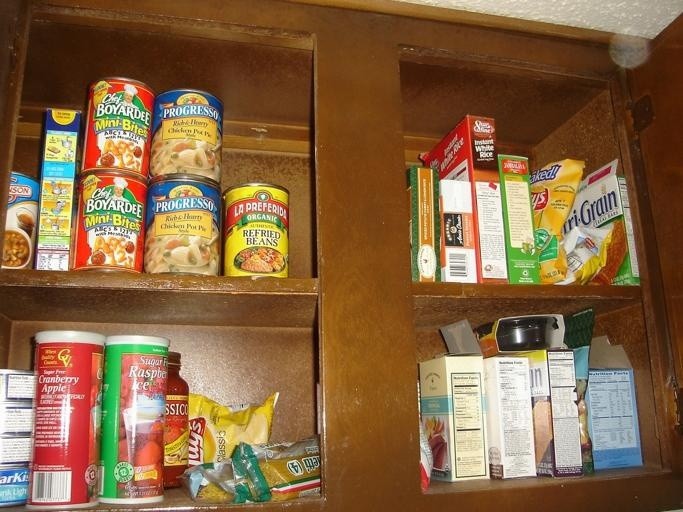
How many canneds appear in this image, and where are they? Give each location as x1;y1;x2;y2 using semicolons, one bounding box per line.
145;177;223;278
149;88;225;183
1;437;33;465
2;404;35;437
107;336;165;503
224;181;289;278
33;330;108;504
0;368;36;401
79;76;154;181
1;466;31;507
73;172;147;274
0;171;41;269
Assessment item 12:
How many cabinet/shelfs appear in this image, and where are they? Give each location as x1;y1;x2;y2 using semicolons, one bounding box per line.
0;0;683;512
